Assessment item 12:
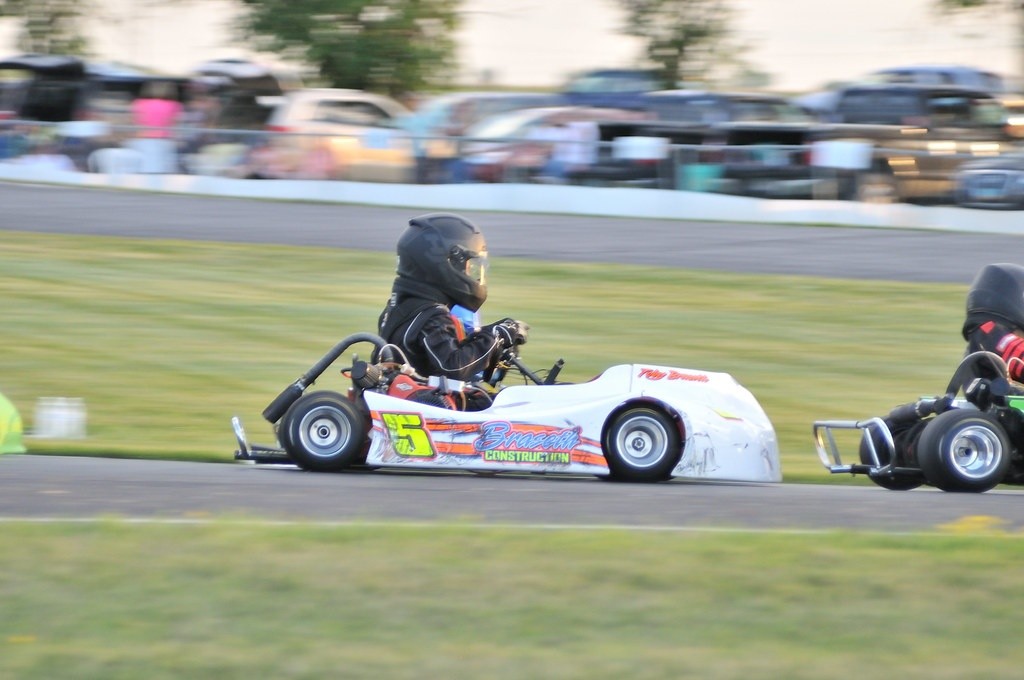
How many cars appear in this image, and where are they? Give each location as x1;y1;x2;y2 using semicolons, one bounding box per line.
0;55;1024;213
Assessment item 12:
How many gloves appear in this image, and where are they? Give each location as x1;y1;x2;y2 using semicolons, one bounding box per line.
481;318;530;349
484;344;519;380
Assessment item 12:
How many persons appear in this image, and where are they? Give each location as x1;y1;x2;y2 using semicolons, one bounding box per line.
131;76;220;174
962;263;1024;385
369;213;530;387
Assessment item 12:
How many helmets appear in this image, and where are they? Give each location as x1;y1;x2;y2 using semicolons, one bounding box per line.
395;212;492;313
966;262;1024;328
